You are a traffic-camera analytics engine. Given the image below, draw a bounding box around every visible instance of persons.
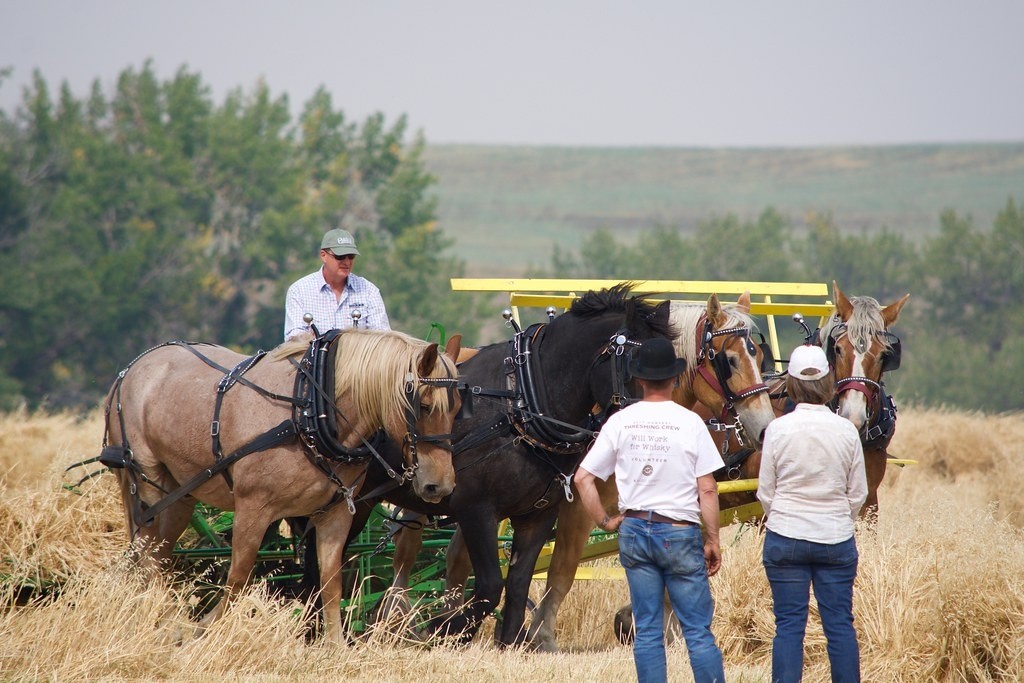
[756,346,868,683]
[285,228,393,343]
[574,339,726,683]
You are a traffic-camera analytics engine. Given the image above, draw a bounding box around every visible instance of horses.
[104,280,912,658]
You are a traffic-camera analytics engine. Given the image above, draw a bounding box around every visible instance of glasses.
[324,250,356,261]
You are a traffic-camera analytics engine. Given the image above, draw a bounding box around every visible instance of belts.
[626,508,695,523]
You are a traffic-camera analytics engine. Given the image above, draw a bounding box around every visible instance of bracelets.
[599,515,610,529]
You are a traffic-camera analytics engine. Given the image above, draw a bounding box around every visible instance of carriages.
[0,278,679,660]
[449,276,919,526]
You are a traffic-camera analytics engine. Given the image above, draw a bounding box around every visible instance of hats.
[787,344,829,381]
[628,339,687,380]
[321,229,361,256]
